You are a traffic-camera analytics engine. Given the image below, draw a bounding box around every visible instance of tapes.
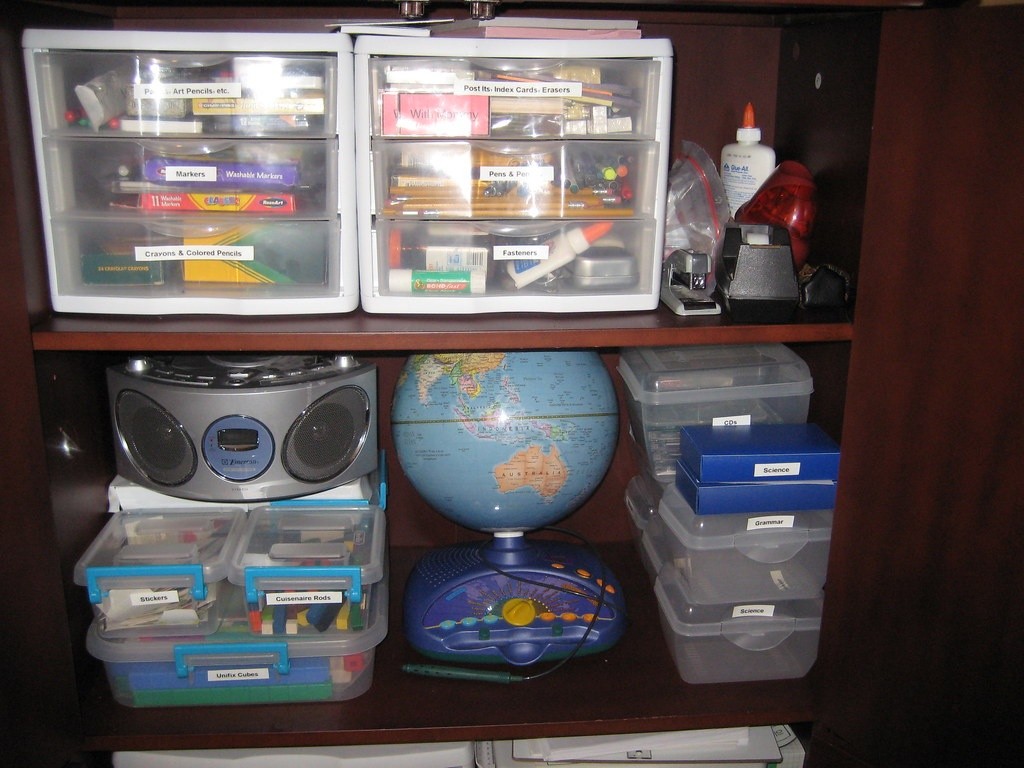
[745,230,770,246]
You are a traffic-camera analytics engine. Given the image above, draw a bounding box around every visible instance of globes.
[389,349,626,667]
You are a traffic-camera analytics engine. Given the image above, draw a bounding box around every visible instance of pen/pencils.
[493,73,614,98]
[483,151,637,205]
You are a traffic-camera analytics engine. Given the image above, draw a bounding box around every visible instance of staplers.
[662,247,724,316]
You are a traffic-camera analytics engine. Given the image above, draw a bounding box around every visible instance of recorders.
[107,353,377,502]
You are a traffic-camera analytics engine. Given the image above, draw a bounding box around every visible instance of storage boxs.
[659,483,832,605]
[370,137,660,220]
[474,723,806,768]
[628,444,670,503]
[354,36,672,316]
[84,566,391,710]
[20,28,358,317]
[44,137,339,220]
[226,507,387,636]
[615,342,815,476]
[674,459,837,514]
[369,55,661,140]
[681,425,840,485]
[73,508,248,637]
[51,220,340,298]
[653,563,827,684]
[377,220,652,298]
[625,478,656,549]
[33,51,336,138]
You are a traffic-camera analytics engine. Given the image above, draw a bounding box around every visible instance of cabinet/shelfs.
[0,0,1024,768]
